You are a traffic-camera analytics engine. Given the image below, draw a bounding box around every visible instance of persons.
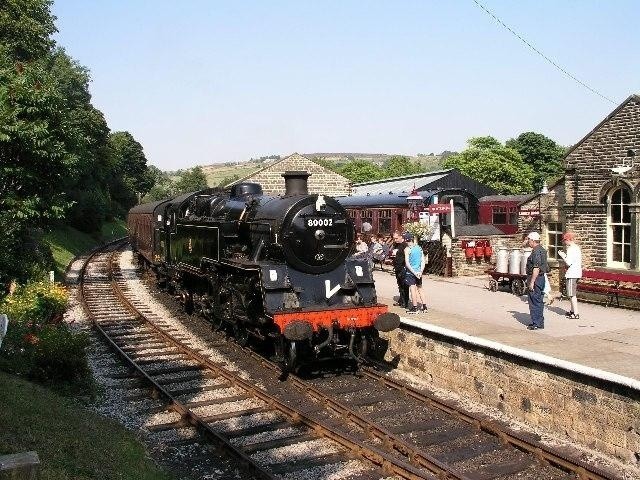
[365,234,387,262]
[356,234,368,258]
[558,232,583,319]
[526,230,548,332]
[404,234,427,314]
[392,231,412,308]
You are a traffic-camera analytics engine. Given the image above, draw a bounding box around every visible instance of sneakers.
[393,302,428,314]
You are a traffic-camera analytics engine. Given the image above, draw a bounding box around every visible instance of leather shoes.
[527,322,544,330]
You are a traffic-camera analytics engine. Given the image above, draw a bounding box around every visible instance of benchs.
[561,267,640,308]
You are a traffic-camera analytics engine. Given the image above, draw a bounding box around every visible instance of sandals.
[565,311,579,319]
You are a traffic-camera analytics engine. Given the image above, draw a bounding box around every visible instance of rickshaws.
[483,268,553,296]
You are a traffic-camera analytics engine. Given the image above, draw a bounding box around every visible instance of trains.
[126,169,402,376]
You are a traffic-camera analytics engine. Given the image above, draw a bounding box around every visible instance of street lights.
[538,181,549,232]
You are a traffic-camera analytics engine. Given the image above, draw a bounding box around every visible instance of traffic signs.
[428,203,451,215]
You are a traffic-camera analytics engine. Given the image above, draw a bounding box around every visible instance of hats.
[560,233,572,242]
[523,232,541,244]
